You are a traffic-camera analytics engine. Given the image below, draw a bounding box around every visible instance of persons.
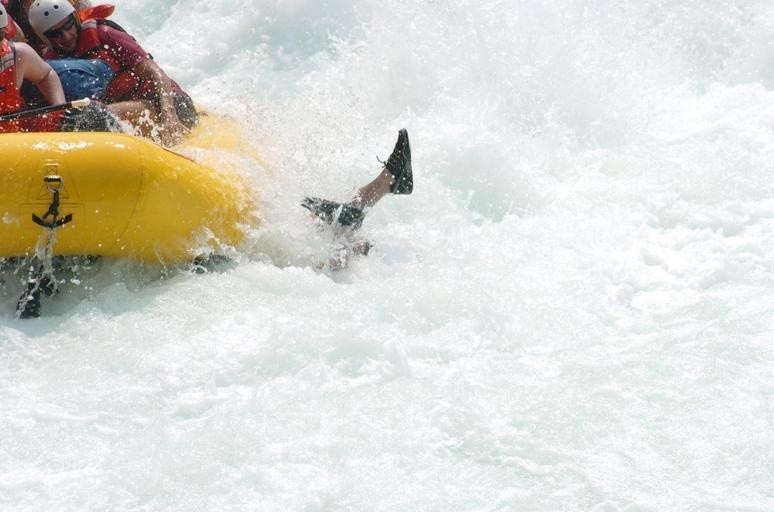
[301,128,414,273]
[0,0,199,148]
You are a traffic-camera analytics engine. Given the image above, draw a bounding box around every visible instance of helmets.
[28,0,79,51]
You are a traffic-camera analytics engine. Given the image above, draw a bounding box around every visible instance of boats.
[0,101,263,267]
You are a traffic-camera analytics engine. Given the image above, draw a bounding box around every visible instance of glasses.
[43,18,76,39]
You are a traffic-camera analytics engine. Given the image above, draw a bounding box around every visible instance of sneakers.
[301,197,364,229]
[381,128,414,195]
